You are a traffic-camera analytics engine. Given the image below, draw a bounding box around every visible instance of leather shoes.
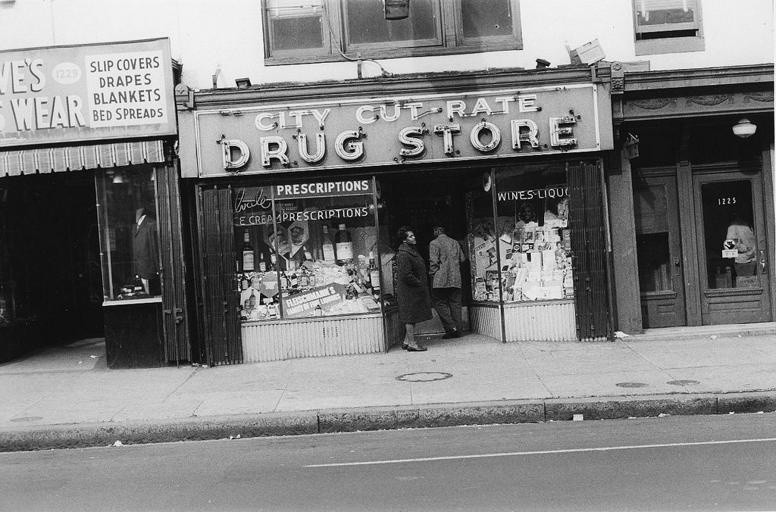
[442,330,465,338]
[402,342,427,351]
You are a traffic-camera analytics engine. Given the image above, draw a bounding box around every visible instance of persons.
[725,202,758,276]
[516,202,539,231]
[543,194,570,229]
[126,207,160,280]
[428,221,468,338]
[393,225,434,351]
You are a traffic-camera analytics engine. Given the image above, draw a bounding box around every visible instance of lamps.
[732,119,757,139]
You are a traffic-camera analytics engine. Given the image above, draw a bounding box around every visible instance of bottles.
[358,250,381,295]
[314,304,322,317]
[249,288,256,307]
[241,272,249,291]
[259,251,267,272]
[267,247,277,265]
[281,268,297,288]
[300,269,308,286]
[302,219,353,265]
[241,225,255,272]
